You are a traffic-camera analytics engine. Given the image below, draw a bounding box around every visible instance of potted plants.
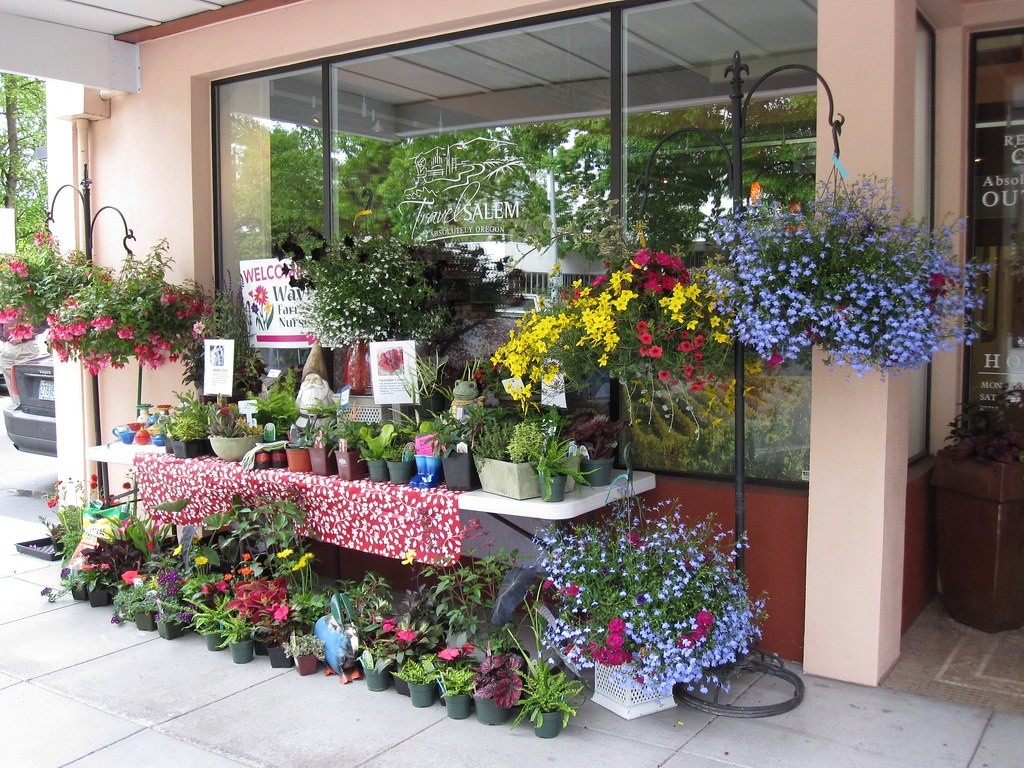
[157,348,633,501]
[934,387,1024,633]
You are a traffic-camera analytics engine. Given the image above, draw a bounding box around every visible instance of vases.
[67,580,677,739]
[0,322,47,346]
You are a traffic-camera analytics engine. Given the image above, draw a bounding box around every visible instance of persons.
[290,373,338,447]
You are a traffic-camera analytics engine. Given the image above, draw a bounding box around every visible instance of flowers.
[38,474,768,697]
[0,172,999,416]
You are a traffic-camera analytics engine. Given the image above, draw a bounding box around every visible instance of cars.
[3,354,57,457]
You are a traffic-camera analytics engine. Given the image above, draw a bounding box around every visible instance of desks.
[82,441,657,683]
